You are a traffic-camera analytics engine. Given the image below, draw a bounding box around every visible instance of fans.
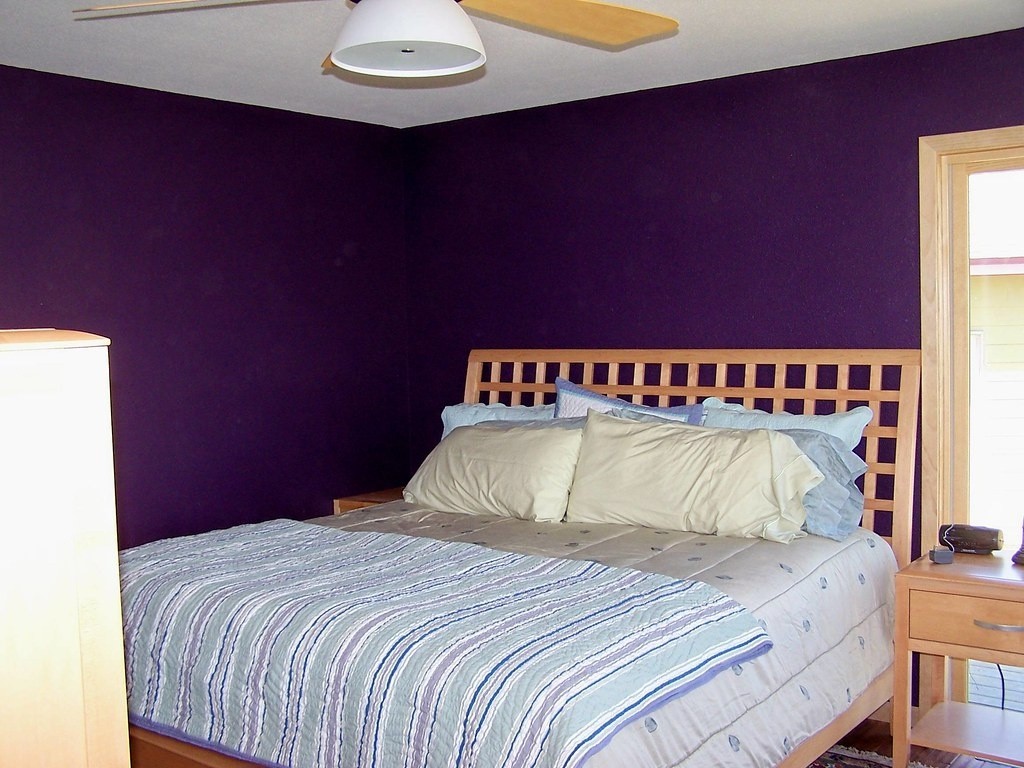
[69,0,682,52]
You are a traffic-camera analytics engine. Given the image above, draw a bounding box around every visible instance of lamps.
[330,0,495,81]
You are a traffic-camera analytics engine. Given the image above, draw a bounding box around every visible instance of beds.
[117,347,920,768]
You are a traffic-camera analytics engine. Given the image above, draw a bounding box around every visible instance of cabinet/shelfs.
[0,328,133,768]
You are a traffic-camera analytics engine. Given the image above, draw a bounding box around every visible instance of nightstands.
[333,488,405,515]
[893,553,1024,768]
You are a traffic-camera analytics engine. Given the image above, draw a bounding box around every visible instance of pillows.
[440,401,554,440]
[478,416,588,431]
[618,408,868,544]
[402,425,583,524]
[703,397,874,453]
[551,377,703,425]
[564,407,822,546]
